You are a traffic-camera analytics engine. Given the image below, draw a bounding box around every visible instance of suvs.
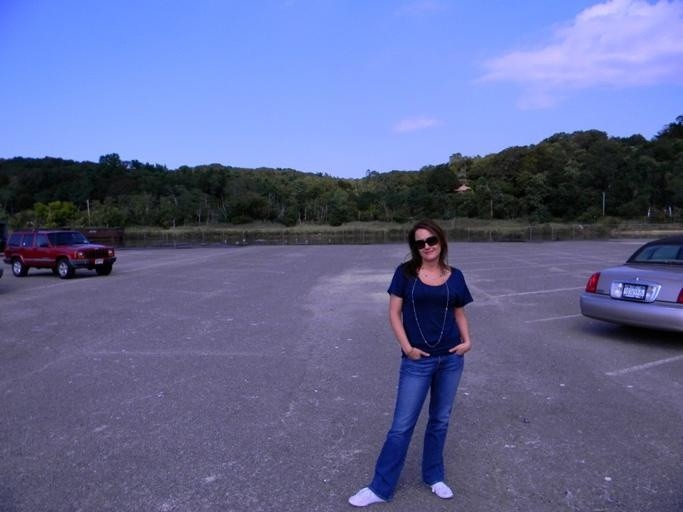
[4,230,117,279]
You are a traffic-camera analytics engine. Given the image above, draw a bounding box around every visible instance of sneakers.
[429,481,454,499]
[348,487,388,507]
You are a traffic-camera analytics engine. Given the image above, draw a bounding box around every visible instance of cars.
[580,235,683,331]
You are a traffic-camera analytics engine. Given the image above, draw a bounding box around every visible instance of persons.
[348,219,474,507]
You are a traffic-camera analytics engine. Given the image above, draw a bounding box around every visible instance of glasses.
[414,236,439,249]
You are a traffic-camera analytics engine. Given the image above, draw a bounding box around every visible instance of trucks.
[0,217,123,253]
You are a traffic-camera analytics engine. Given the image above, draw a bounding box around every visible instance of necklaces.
[419,269,445,281]
